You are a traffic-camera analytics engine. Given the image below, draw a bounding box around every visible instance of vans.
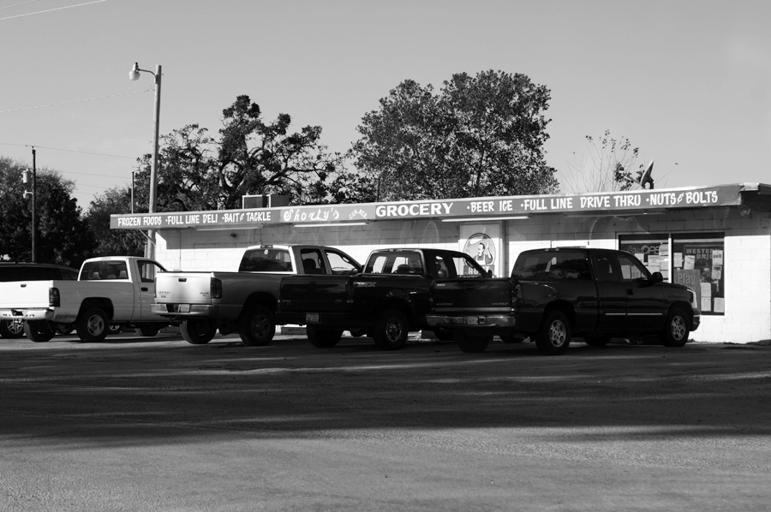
[0,262,85,336]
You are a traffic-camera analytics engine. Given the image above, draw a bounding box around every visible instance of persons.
[472,242,491,265]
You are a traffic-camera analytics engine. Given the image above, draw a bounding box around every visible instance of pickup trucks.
[150,240,366,345]
[275,247,503,352]
[0,255,180,342]
[425,243,705,353]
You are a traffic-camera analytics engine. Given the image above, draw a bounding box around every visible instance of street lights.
[126,59,166,279]
[19,167,38,262]
